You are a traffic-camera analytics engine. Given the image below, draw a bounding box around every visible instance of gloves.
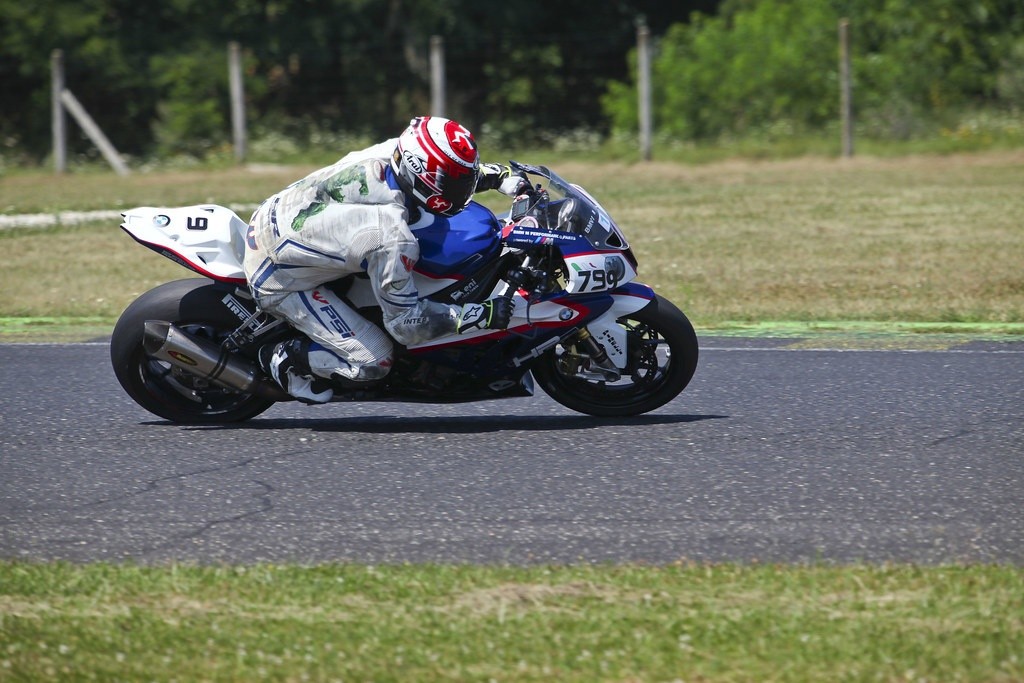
[476,163,534,199]
[456,296,515,334]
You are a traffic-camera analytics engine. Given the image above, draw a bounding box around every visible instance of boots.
[258,336,333,405]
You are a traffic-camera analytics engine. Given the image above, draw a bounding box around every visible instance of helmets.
[390,116,481,218]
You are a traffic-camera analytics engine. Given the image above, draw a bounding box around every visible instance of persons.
[242,117,535,415]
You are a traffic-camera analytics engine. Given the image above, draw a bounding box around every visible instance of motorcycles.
[108,159,701,421]
